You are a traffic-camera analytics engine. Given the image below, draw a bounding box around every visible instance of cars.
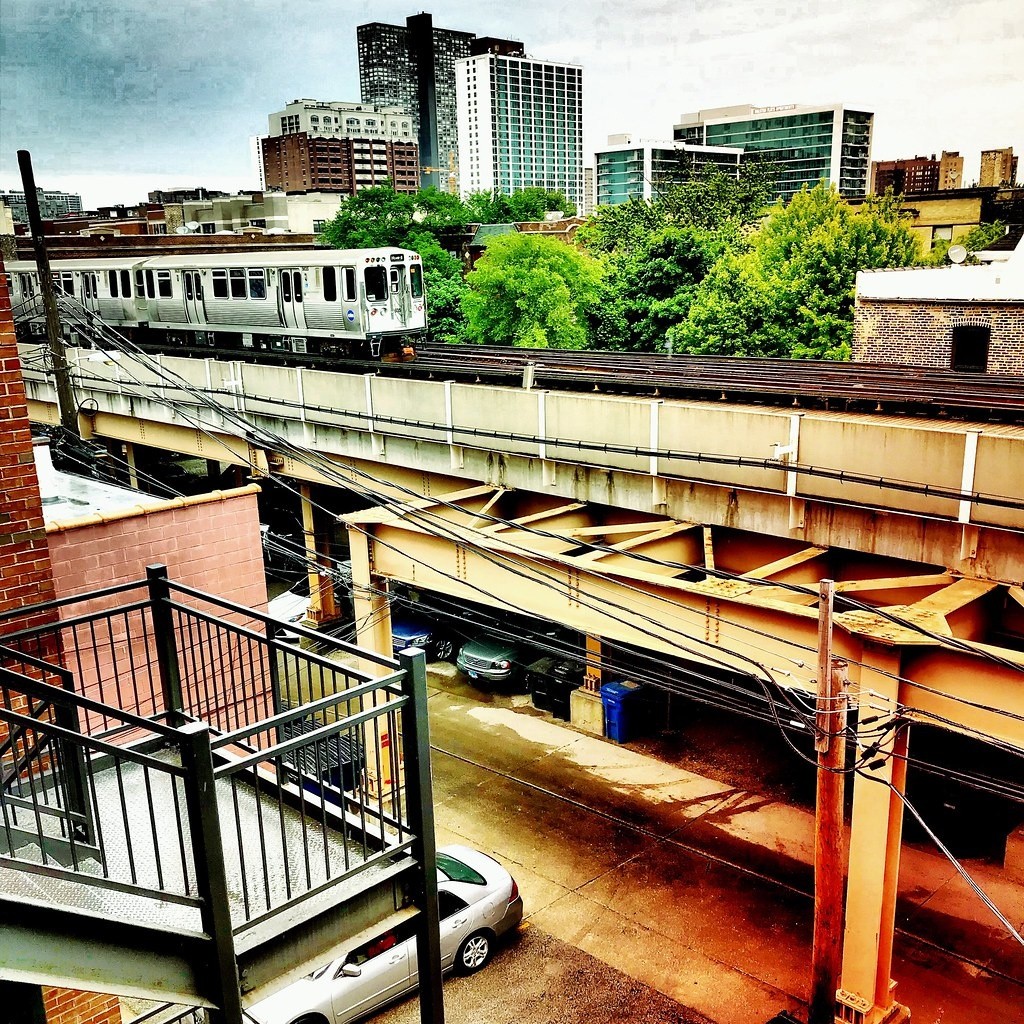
[268,561,351,640]
[266,559,586,722]
[241,845,524,1024]
[392,617,466,662]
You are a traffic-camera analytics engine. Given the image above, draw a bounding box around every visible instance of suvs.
[457,634,542,694]
[524,647,596,715]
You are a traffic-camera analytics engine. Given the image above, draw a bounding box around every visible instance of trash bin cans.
[598,678,647,745]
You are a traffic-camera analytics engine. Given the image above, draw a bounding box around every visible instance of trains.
[0,247,427,355]
